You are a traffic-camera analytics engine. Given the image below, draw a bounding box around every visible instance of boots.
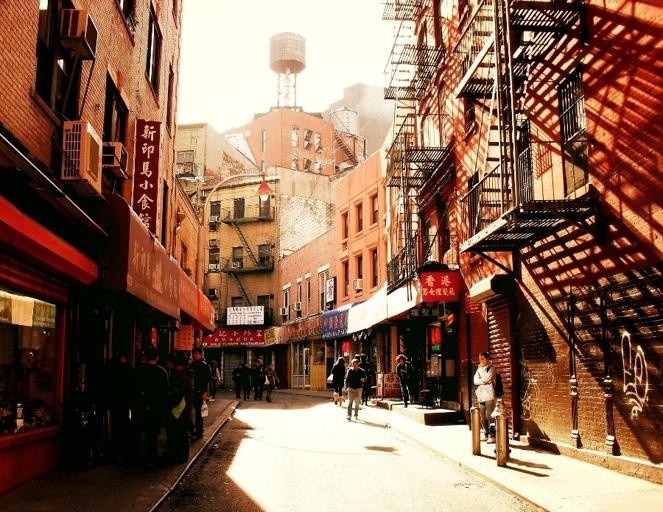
[338,396,345,406]
[334,392,338,404]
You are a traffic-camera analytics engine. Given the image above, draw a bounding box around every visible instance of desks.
[375,372,401,400]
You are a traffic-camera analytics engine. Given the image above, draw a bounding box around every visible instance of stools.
[420,389,432,408]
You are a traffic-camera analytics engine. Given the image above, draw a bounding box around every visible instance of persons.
[92,346,221,476]
[396,354,417,407]
[232,359,279,403]
[331,354,374,421]
[474,351,498,443]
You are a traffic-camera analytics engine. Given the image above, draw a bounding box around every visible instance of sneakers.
[487,437,496,443]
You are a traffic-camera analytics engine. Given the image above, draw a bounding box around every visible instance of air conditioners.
[60,9,98,60]
[294,302,301,311]
[61,121,103,196]
[280,307,288,316]
[207,216,240,297]
[353,279,363,291]
[103,142,129,180]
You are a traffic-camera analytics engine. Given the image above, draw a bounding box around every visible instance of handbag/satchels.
[327,374,333,384]
[494,373,504,397]
